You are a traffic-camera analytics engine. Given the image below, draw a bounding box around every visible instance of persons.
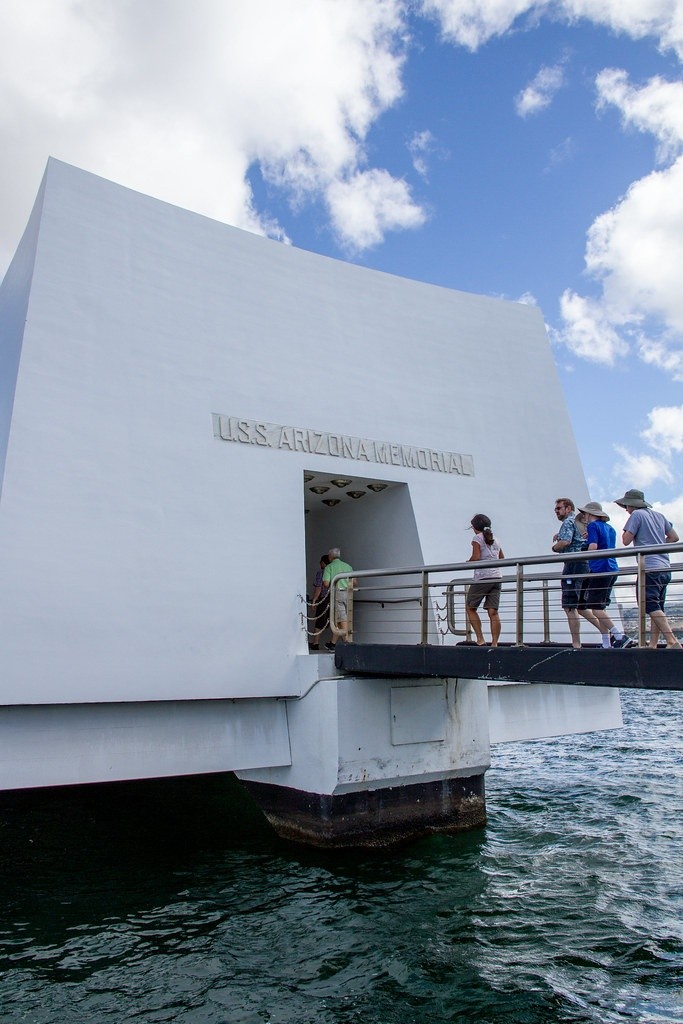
[552,498,633,649]
[466,515,505,647]
[614,489,683,649]
[309,548,357,653]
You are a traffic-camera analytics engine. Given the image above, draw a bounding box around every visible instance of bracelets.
[553,547,557,552]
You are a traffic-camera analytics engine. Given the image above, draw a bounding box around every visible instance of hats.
[321,554,330,564]
[308,642,319,650]
[614,489,653,508]
[577,502,610,521]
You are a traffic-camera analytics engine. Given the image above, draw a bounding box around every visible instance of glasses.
[555,506,567,510]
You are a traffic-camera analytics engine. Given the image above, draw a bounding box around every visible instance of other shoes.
[609,635,632,648]
[570,645,583,649]
[665,641,683,649]
[596,645,612,649]
[470,642,487,646]
[324,641,336,653]
